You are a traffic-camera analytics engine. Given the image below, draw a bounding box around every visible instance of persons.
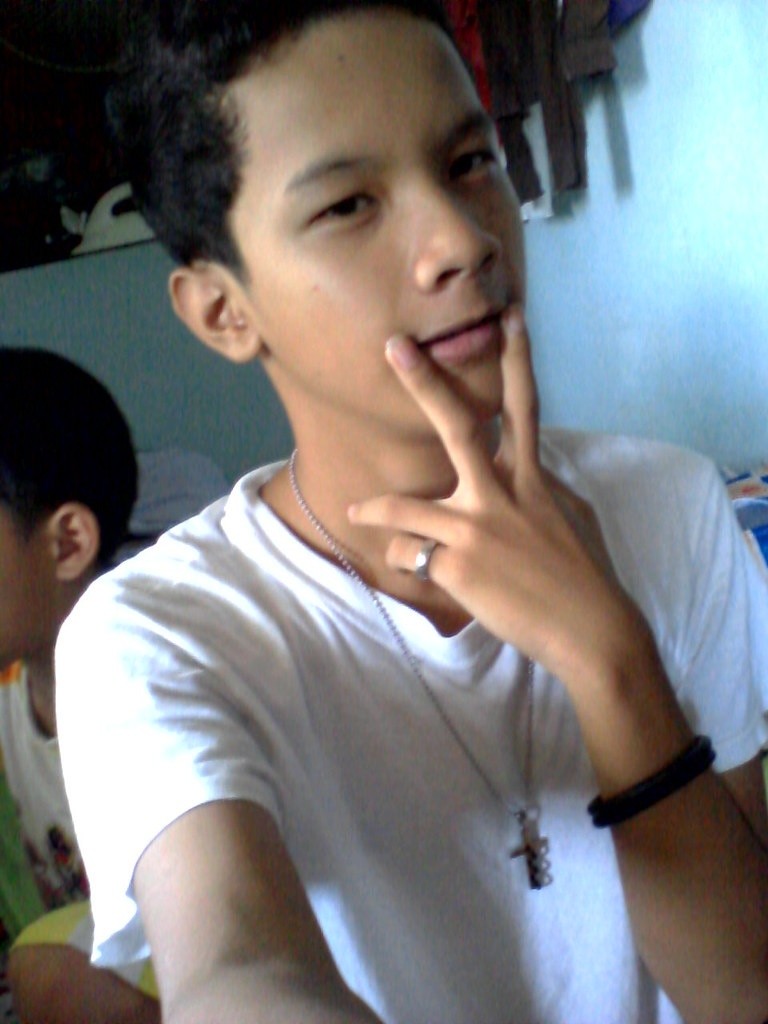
[0,346,160,1024]
[51,0,766,1024]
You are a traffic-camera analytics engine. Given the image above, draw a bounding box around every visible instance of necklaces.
[290,447,538,890]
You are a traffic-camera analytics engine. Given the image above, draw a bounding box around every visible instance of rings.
[415,541,436,582]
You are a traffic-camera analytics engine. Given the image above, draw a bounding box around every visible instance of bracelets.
[587,737,715,828]
